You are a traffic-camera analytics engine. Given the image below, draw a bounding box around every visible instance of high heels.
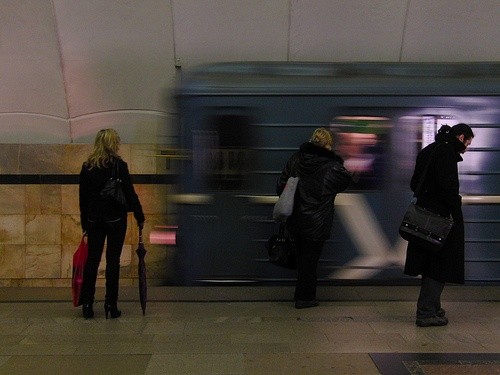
[105,303,122,318]
[83,304,94,320]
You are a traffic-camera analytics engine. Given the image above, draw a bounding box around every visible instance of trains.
[169,61,500,285]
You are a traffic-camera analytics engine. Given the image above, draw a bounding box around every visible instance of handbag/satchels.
[399,201,454,251]
[273,152,301,218]
[266,217,294,268]
[72,233,89,306]
[100,157,136,212]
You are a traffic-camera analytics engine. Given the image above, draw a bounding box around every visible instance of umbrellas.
[136,224,147,315]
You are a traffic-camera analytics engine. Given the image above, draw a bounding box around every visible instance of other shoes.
[437,309,446,316]
[416,315,448,327]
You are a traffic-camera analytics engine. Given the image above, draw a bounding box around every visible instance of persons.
[79,128,146,320]
[403,123,474,326]
[280,127,351,309]
